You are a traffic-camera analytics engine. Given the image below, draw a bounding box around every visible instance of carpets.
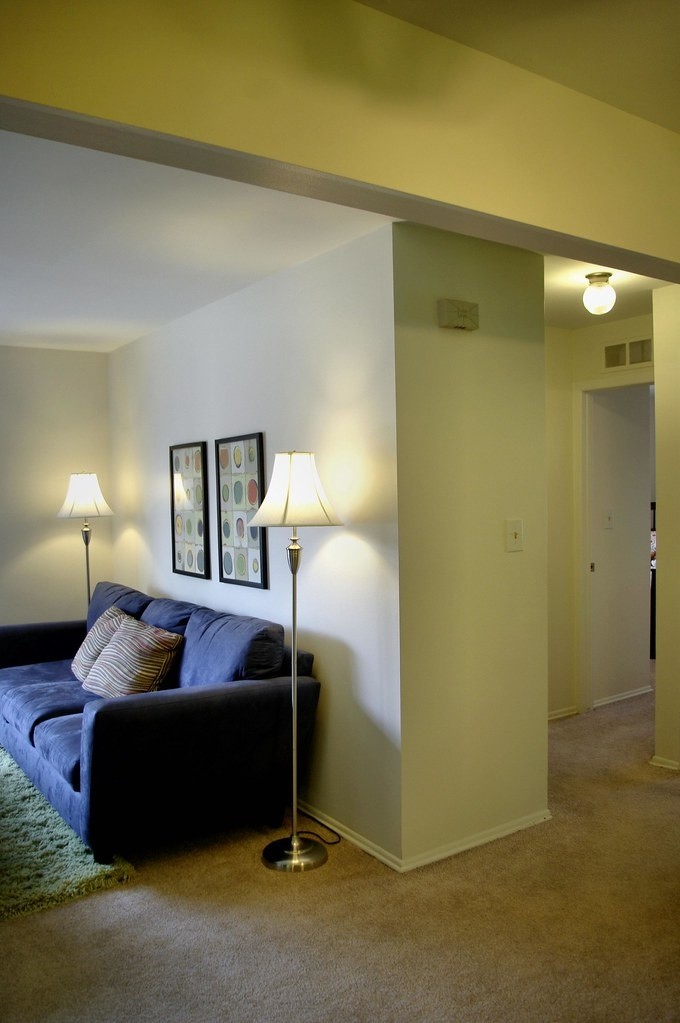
[0,744,141,922]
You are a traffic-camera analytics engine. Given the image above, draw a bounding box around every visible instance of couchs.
[0,581,321,868]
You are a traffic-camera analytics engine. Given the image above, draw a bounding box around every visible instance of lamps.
[247,450,344,871]
[582,272,617,315]
[55,471,115,605]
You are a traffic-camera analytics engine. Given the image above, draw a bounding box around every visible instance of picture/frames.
[84,615,183,699]
[215,432,269,590]
[168,441,211,580]
[71,605,127,683]
